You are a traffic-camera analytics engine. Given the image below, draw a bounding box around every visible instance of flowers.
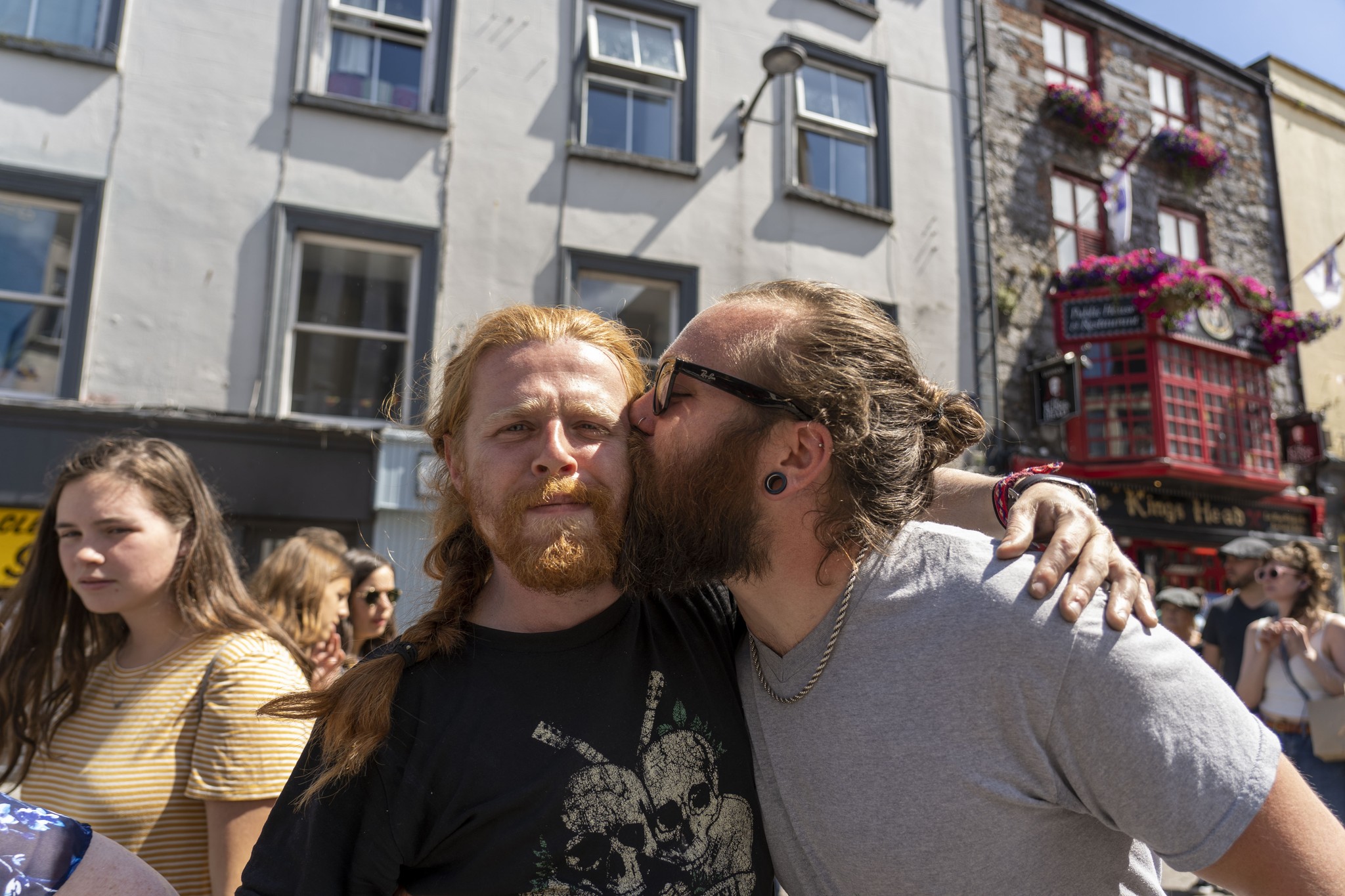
[1043,81,1124,148]
[1053,247,1337,358]
[1155,123,1229,179]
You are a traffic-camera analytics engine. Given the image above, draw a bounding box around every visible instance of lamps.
[734,43,802,158]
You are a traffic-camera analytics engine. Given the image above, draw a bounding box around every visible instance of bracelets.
[1009,473,1098,515]
[992,462,1063,552]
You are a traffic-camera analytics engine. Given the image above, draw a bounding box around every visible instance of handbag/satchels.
[1309,694,1345,762]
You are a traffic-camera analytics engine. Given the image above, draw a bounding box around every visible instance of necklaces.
[750,538,868,704]
[110,622,188,709]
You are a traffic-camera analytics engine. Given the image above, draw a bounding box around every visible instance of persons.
[625,279,1345,896]
[0,438,317,896]
[1138,537,1345,831]
[233,309,1164,896]
[242,526,399,691]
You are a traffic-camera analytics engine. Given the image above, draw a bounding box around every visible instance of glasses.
[653,359,829,421]
[1254,565,1304,584]
[365,590,398,604]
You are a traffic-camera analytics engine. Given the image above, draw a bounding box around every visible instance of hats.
[1155,587,1200,615]
[1220,536,1272,559]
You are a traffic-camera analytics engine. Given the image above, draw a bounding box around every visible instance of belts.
[1262,721,1310,733]
